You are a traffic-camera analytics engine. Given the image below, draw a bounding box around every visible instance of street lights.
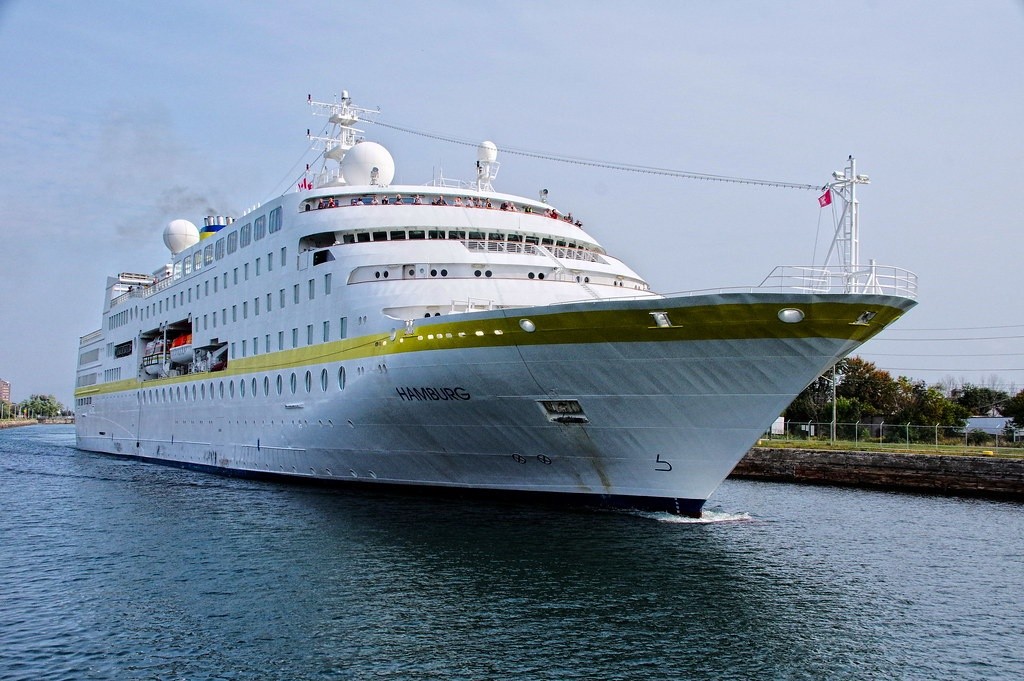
[2,402,36,419]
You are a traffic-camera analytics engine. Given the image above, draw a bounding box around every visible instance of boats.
[73,88,921,519]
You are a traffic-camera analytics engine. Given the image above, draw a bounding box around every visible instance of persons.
[382,195,389,205]
[318,198,325,209]
[127,281,156,292]
[575,219,582,228]
[394,193,404,205]
[432,196,448,206]
[455,196,493,209]
[500,200,532,214]
[542,209,558,220]
[327,197,336,208]
[352,198,364,206]
[371,196,378,205]
[562,212,573,224]
[412,194,423,205]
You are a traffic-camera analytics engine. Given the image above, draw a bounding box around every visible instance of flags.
[818,188,832,208]
[298,178,312,190]
